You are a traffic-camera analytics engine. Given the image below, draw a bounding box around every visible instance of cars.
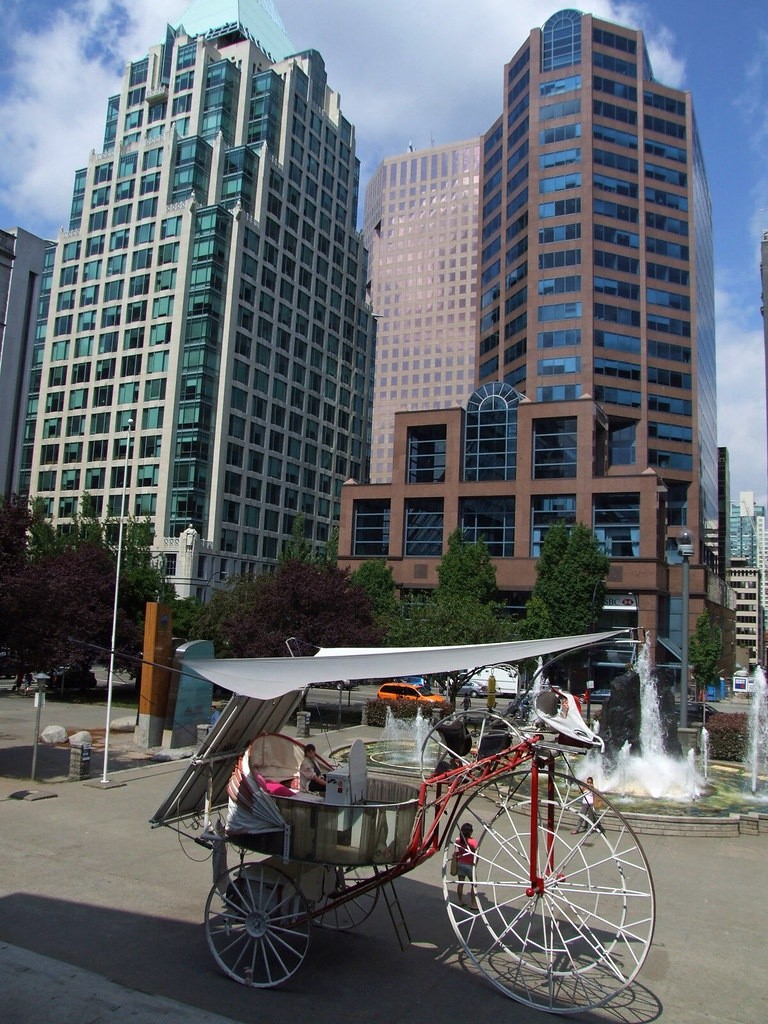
[676,701,721,723]
[310,679,360,691]
[438,681,489,698]
[518,684,562,702]
[578,689,611,705]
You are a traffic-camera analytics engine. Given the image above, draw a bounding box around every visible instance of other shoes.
[571,830,578,834]
[470,906,484,910]
[458,902,467,907]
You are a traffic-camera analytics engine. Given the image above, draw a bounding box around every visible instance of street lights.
[676,527,696,730]
[202,570,227,605]
[30,672,52,782]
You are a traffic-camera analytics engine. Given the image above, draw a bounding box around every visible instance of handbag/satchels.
[450,841,459,876]
[594,794,602,808]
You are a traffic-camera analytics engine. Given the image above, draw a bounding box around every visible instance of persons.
[454,823,484,910]
[460,693,472,711]
[298,744,342,793]
[209,706,220,725]
[15,670,34,696]
[572,776,596,835]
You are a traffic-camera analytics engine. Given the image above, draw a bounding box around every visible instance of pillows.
[266,779,296,796]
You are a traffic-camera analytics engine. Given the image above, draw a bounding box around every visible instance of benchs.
[260,776,324,803]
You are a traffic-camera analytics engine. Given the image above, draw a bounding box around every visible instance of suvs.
[403,677,432,691]
[377,683,447,705]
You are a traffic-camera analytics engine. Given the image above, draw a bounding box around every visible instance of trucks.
[458,663,519,698]
